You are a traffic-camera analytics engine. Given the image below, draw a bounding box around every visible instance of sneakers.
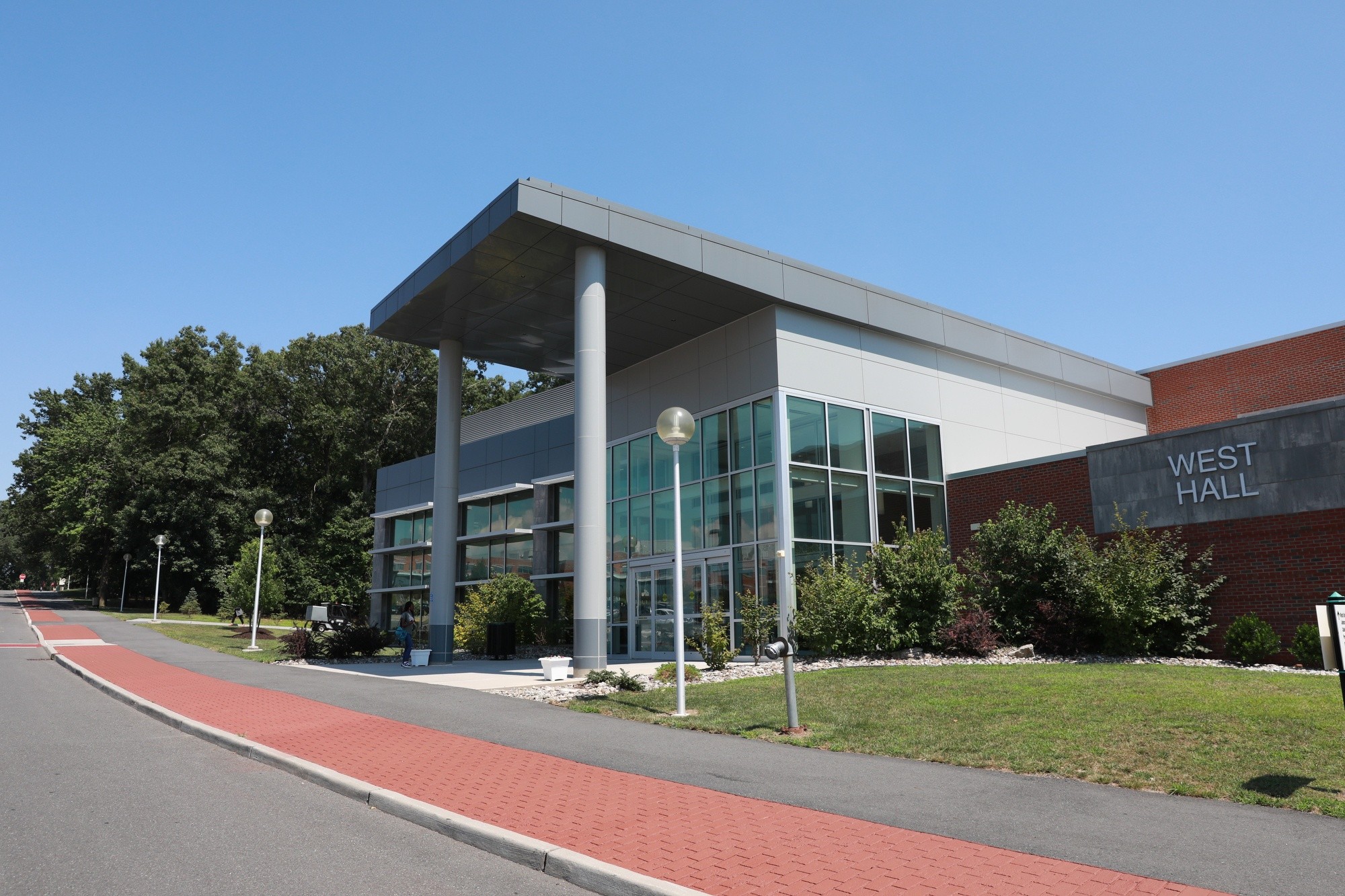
[401,662,412,668]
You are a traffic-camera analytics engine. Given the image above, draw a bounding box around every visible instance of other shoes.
[242,624,245,627]
[229,623,233,626]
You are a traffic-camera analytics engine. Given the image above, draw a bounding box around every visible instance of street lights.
[120,554,131,612]
[152,535,167,621]
[242,509,274,651]
[656,406,696,717]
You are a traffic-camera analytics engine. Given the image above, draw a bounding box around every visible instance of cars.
[654,608,693,631]
[607,608,619,618]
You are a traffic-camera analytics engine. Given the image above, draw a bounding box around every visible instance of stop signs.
[20,574,26,579]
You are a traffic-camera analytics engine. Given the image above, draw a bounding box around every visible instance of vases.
[538,655,572,681]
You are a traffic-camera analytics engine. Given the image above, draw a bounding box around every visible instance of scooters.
[306,602,353,632]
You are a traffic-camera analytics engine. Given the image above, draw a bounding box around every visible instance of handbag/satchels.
[51,583,54,587]
[235,608,244,616]
[395,626,408,642]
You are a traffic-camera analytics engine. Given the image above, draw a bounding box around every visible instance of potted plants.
[410,622,433,667]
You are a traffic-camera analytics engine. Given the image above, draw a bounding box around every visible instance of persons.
[400,601,415,668]
